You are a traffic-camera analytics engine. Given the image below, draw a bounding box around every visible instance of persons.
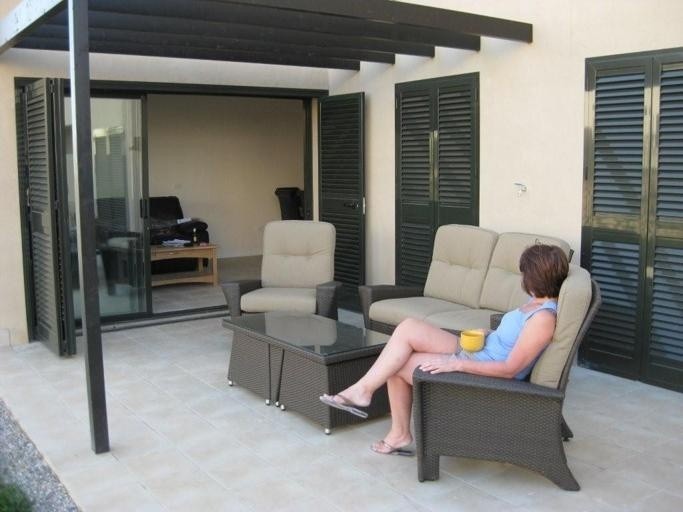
[316,242,568,455]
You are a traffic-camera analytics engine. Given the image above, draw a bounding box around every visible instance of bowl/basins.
[460,330,485,352]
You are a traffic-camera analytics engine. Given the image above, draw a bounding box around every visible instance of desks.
[150,245,220,287]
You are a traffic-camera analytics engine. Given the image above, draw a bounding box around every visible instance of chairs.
[222,220,345,319]
[95,195,210,295]
[409,263,603,491]
[274,186,304,219]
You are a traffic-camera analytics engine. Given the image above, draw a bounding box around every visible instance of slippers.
[371,440,415,456]
[319,393,369,419]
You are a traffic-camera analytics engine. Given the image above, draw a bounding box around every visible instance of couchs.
[358,222,574,339]
